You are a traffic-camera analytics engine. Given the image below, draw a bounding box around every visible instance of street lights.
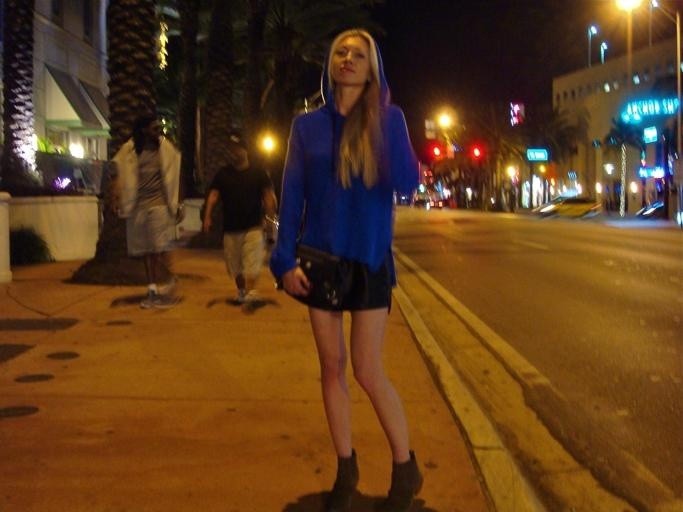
[615,0,642,98]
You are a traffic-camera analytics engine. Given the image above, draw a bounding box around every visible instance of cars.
[533,195,601,217]
[638,202,666,218]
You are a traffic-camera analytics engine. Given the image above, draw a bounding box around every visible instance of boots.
[326,447,358,512]
[384,450,423,512]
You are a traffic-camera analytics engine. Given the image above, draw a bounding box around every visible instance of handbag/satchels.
[279,243,352,309]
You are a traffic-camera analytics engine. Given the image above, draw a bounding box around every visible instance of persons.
[204,133,278,302]
[268,29,423,512]
[109,113,186,309]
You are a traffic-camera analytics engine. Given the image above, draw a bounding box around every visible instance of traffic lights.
[438,111,455,129]
[431,142,445,160]
[469,144,484,159]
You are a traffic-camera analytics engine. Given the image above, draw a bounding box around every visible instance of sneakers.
[238,288,261,304]
[141,276,183,308]
